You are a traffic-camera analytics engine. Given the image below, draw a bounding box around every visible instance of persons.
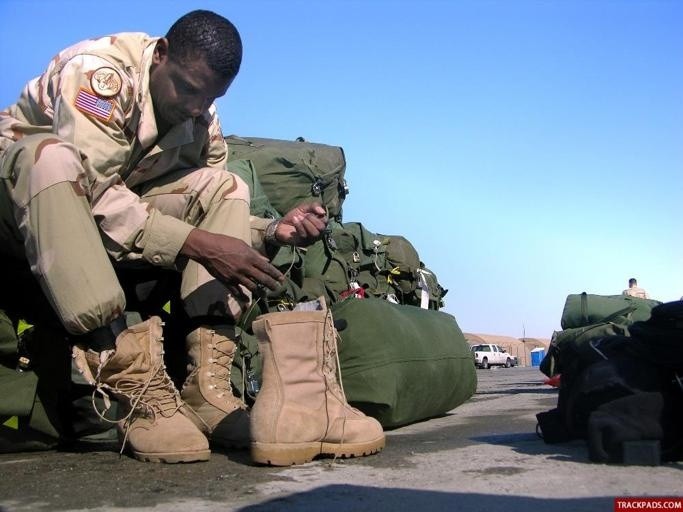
[0,9,329,464]
[622,278,649,300]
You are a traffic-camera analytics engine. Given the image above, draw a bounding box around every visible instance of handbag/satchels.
[537,292,682,464]
[224,135,478,425]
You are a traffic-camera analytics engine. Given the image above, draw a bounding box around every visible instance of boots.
[70,316,212,464]
[248,310,386,466]
[181,326,252,446]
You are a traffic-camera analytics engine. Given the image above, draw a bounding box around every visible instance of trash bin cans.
[531,351,545,367]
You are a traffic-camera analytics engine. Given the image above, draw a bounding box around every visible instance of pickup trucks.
[471,344,512,369]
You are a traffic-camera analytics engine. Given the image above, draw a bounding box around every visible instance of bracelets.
[265,219,282,247]
